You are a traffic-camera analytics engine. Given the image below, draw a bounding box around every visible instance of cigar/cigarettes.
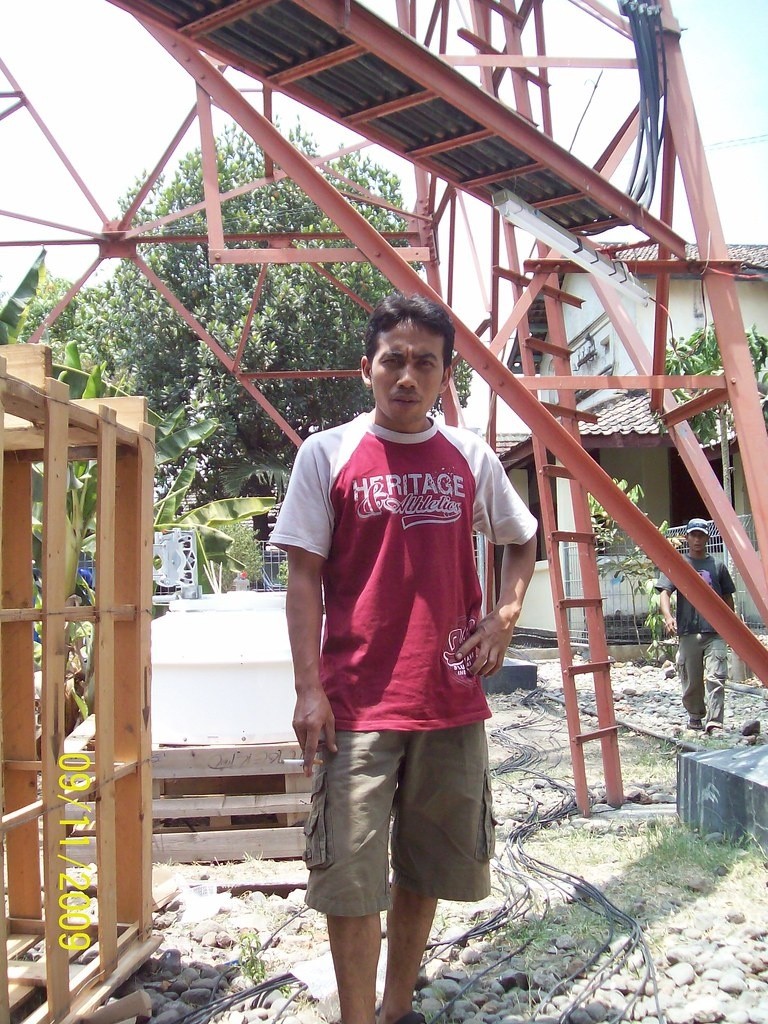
[278,758,323,766]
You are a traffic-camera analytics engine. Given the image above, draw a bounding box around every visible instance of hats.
[686,518,710,535]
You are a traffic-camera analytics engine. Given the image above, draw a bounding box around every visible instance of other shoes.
[688,718,703,729]
[709,726,725,739]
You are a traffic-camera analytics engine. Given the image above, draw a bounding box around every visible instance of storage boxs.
[61,712,317,872]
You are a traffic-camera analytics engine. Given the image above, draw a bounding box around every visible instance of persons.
[655,519,737,740]
[269,292,538,1024]
[249,471,285,588]
